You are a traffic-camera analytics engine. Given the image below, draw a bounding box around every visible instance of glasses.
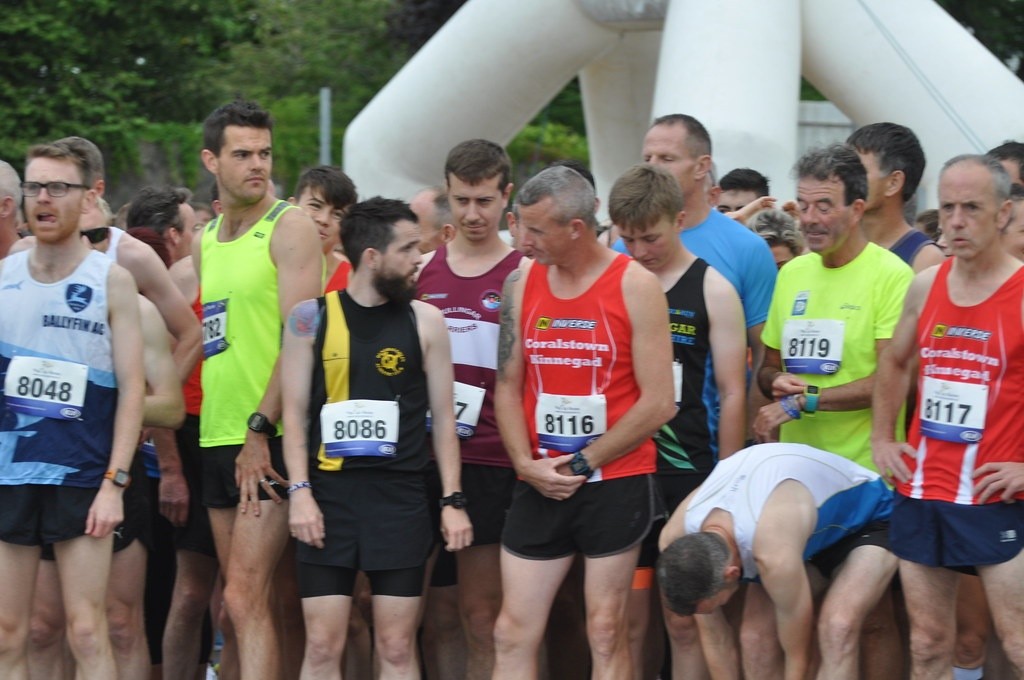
[20,182,90,197]
[80,227,110,243]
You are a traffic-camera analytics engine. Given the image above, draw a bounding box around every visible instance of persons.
[0,102,1024,680]
